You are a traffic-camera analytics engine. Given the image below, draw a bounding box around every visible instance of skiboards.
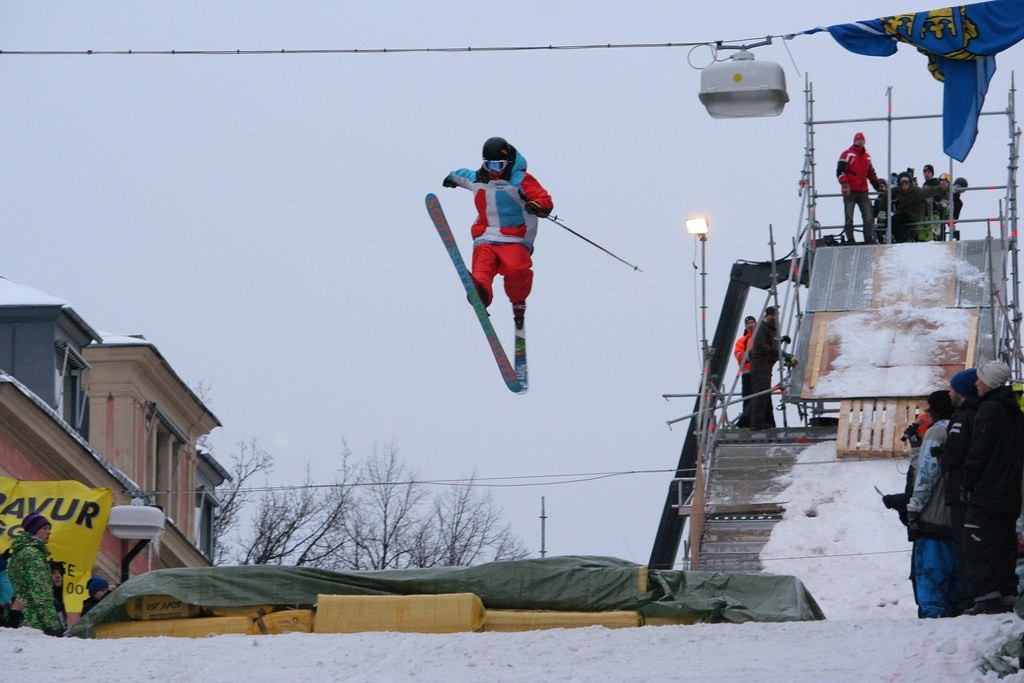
[425,192,529,395]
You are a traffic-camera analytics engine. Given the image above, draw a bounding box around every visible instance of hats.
[86,576,108,590]
[914,361,1011,433]
[854,132,865,140]
[745,316,754,322]
[22,511,52,535]
[893,164,967,193]
[766,306,779,316]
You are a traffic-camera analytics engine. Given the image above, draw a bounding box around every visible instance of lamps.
[698,40,790,122]
[105,492,166,543]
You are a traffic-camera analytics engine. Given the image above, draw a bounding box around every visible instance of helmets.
[482,137,509,159]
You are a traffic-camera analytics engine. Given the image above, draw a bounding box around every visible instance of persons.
[837,132,884,245]
[882,360,1024,621]
[735,306,799,431]
[442,137,554,330]
[0,512,116,638]
[872,165,968,244]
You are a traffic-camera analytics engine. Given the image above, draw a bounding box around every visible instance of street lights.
[685,217,710,381]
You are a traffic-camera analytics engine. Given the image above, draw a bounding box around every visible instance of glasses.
[88,589,99,596]
[482,160,507,171]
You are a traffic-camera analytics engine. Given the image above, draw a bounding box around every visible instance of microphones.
[900,417,924,442]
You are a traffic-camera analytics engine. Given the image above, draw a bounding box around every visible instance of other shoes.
[512,301,526,317]
[937,585,1018,614]
[467,286,488,309]
[848,238,878,246]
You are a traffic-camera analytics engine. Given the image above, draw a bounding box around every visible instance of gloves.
[944,483,971,505]
[842,183,851,198]
[524,200,541,214]
[900,511,920,530]
[883,495,896,509]
[904,423,922,447]
[44,627,64,638]
[442,176,457,189]
